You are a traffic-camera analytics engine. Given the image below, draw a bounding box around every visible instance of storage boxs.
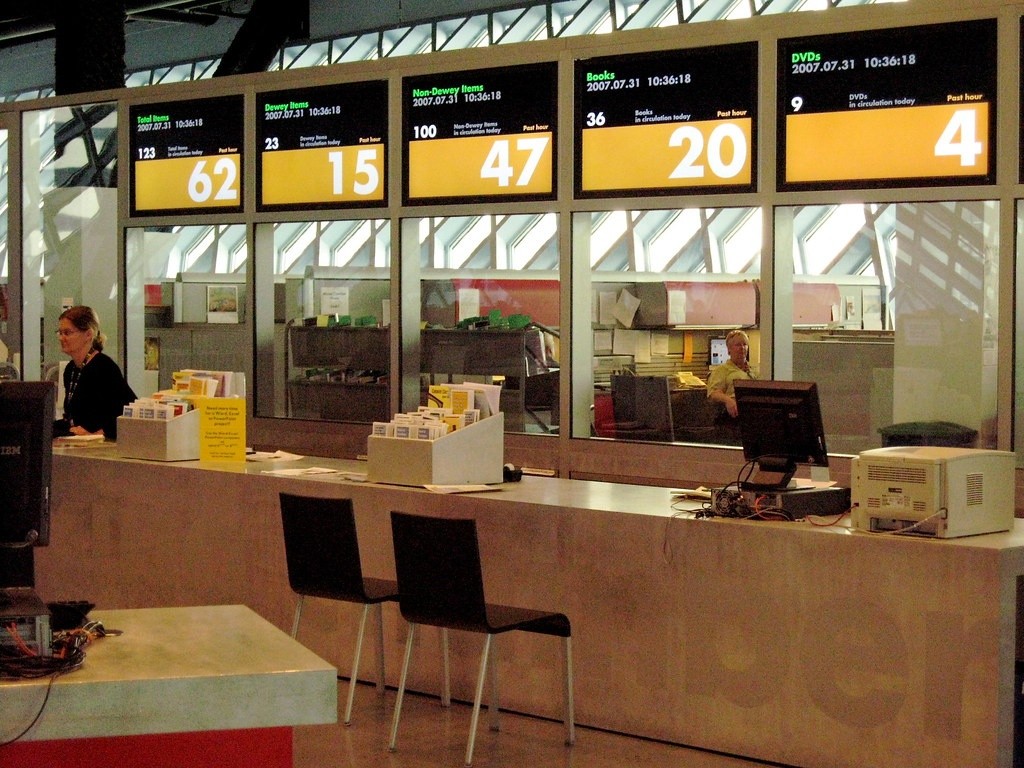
[366,412,503,486]
[115,407,198,463]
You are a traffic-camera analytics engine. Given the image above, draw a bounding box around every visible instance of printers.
[850,446,1015,539]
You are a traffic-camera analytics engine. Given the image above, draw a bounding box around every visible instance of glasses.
[55,328,81,336]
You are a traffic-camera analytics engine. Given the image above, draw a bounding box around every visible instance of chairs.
[387,509,575,764]
[277,490,452,728]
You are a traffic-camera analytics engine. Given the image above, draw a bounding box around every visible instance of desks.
[0,603,338,768]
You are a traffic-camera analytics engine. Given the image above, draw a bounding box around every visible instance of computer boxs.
[711,484,851,521]
[0,588,53,660]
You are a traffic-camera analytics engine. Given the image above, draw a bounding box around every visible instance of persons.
[708,330,763,418]
[53,305,138,444]
[541,331,560,427]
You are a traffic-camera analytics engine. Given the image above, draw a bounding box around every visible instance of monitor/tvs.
[0,381,55,611]
[708,335,749,367]
[734,378,829,492]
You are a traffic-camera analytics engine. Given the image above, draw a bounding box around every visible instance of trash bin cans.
[877,419,981,447]
